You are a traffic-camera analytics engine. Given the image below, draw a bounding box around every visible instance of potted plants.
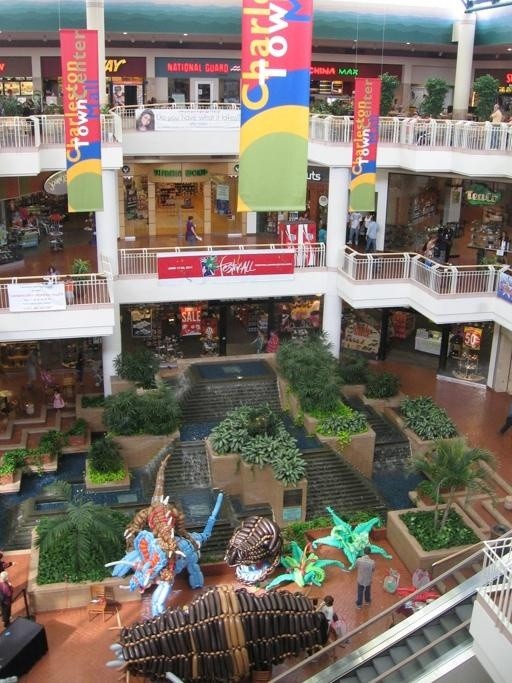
[71,257,92,298]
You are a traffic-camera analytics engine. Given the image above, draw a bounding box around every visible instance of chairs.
[87,583,110,623]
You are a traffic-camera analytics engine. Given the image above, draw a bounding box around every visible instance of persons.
[64,273,75,304]
[51,389,65,414]
[0,552,15,574]
[135,108,155,132]
[74,351,86,387]
[22,348,43,392]
[489,103,504,123]
[497,230,509,265]
[353,545,376,609]
[44,368,57,388]
[44,264,60,283]
[329,614,351,648]
[493,405,512,436]
[0,571,14,628]
[185,215,202,251]
[95,365,104,387]
[249,328,265,354]
[315,595,335,623]
[264,331,280,353]
[298,209,455,261]
